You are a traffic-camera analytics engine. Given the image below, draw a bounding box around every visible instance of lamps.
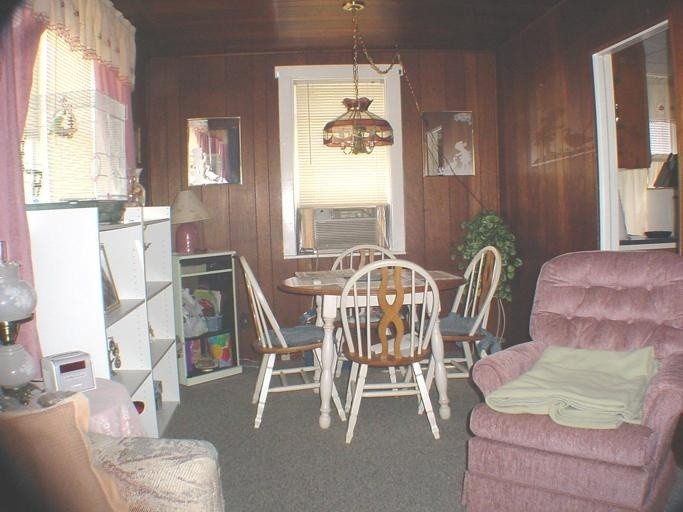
[321,1,394,156]
[0,237,39,406]
[168,189,212,255]
[652,152,680,239]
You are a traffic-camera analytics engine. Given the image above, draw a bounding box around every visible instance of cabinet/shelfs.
[174,249,242,387]
[26,205,184,440]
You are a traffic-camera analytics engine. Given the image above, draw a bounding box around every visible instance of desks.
[0,374,145,438]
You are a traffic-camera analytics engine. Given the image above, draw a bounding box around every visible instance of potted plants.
[450,210,523,304]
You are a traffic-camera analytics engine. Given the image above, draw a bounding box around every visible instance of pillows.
[1,392,131,511]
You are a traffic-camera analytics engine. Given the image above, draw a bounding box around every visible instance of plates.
[645,230,672,239]
[36,390,74,407]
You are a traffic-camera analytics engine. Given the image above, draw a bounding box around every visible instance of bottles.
[124,168,146,206]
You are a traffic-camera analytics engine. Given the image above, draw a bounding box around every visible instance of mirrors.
[184,115,242,188]
[420,109,475,178]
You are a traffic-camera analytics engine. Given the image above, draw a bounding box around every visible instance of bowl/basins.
[77,199,128,224]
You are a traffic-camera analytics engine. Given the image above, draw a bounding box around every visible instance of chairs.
[241,244,502,443]
[462,248,681,512]
[0,394,226,511]
